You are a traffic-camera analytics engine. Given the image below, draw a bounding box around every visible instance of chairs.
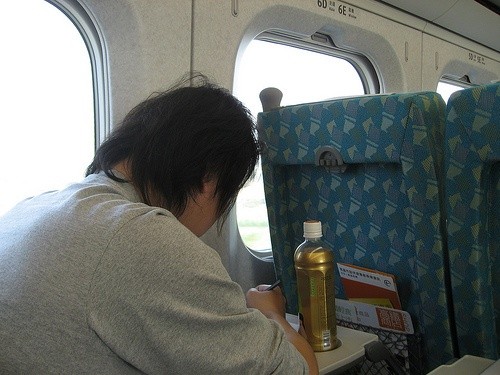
[445,77,500,360]
[256,89,456,374]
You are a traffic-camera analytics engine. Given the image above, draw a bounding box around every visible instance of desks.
[423,353,499,375]
[284,312,407,375]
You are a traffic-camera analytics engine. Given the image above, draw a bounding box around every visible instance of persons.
[0,69,320,375]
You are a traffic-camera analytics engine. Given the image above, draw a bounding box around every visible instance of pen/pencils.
[265,279,282,290]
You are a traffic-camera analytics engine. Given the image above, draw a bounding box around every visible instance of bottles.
[294,220,338,351]
